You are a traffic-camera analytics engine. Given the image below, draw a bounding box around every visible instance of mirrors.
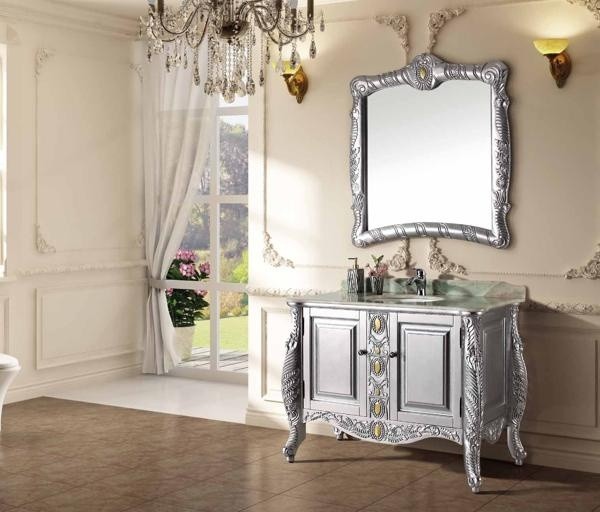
[349,52,513,250]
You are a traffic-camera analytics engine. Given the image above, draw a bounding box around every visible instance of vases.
[165,326,195,362]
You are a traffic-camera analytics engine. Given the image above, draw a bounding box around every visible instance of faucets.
[408,267,427,297]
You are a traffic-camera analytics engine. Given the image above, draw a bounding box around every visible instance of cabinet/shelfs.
[299,303,514,430]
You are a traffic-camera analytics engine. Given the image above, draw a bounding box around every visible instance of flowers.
[165,248,211,327]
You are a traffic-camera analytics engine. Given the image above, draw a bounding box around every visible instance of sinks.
[363,293,445,304]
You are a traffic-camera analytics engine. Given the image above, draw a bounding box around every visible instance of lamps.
[271,57,308,104]
[532,39,571,88]
[136,0,325,103]
